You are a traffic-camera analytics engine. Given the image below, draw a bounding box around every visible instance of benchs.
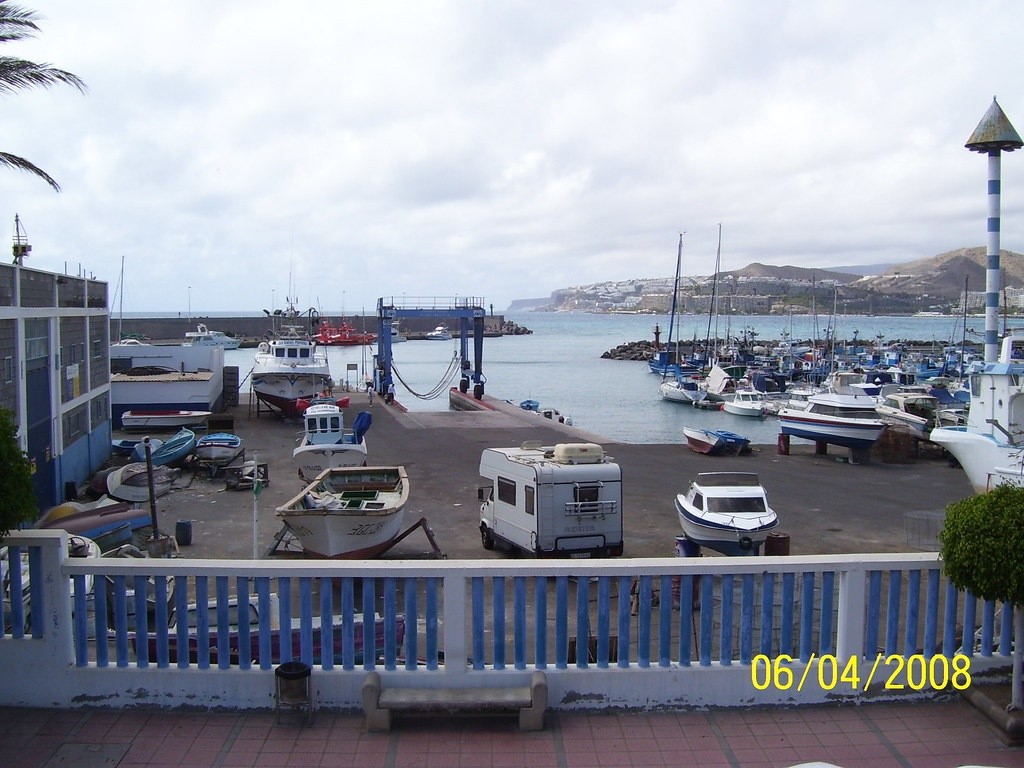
[361,671,548,732]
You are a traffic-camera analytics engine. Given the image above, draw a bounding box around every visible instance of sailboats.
[656,222,985,417]
[108,254,149,346]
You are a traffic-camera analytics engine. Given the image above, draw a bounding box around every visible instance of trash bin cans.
[65,480,77,502]
[278,662,309,705]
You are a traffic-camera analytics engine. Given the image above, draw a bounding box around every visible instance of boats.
[181,324,243,350]
[195,433,241,467]
[504,397,572,427]
[777,288,892,449]
[121,409,213,428]
[425,321,452,340]
[276,464,409,558]
[293,369,368,485]
[874,389,971,443]
[133,589,404,664]
[682,425,751,456]
[649,347,700,378]
[251,226,330,413]
[675,471,780,557]
[0,426,195,620]
[316,290,376,345]
[389,320,407,343]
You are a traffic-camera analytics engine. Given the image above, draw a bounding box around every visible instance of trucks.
[476,440,622,559]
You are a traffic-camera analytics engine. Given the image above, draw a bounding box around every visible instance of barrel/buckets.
[675,536,700,557]
[176,520,192,544]
[764,531,790,556]
[778,433,790,454]
[816,441,827,454]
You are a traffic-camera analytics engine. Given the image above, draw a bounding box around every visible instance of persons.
[367,383,374,408]
[388,384,396,405]
